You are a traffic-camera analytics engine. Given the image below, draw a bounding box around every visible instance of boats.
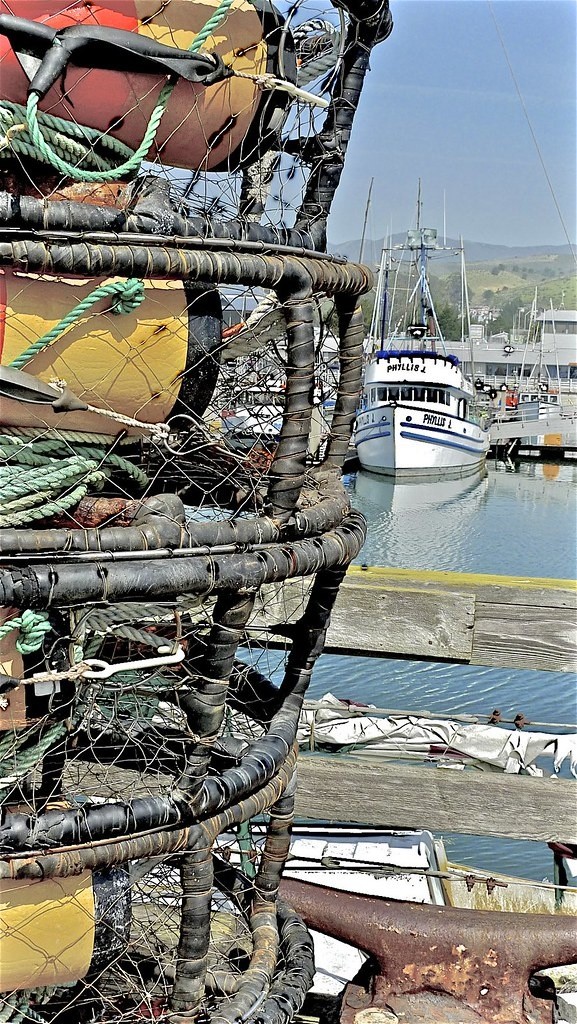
[352,176,493,479]
[0,0,577,1024]
